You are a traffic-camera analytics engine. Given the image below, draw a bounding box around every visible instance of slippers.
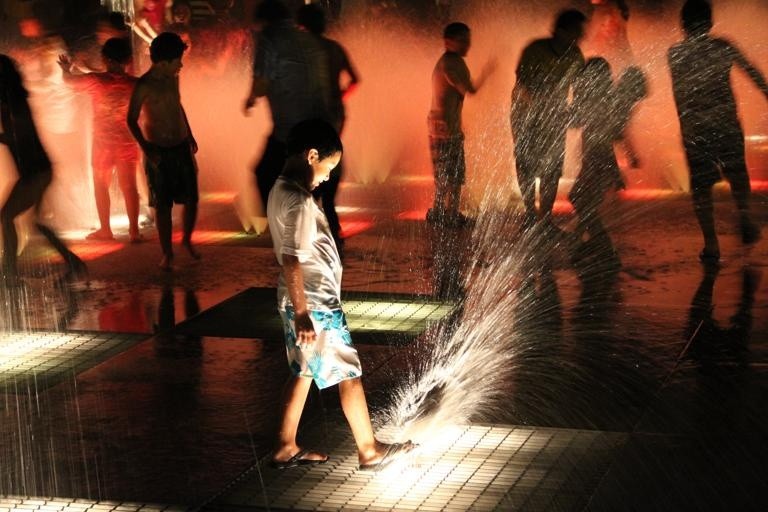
[269,446,331,472]
[358,439,421,473]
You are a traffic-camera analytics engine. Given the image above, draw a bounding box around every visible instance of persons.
[582,0,641,170]
[664,0,766,263]
[424,21,500,230]
[295,2,360,140]
[240,0,343,263]
[507,5,592,220]
[0,1,265,134]
[126,32,201,272]
[264,118,420,473]
[0,52,88,292]
[559,55,643,253]
[56,36,145,243]
